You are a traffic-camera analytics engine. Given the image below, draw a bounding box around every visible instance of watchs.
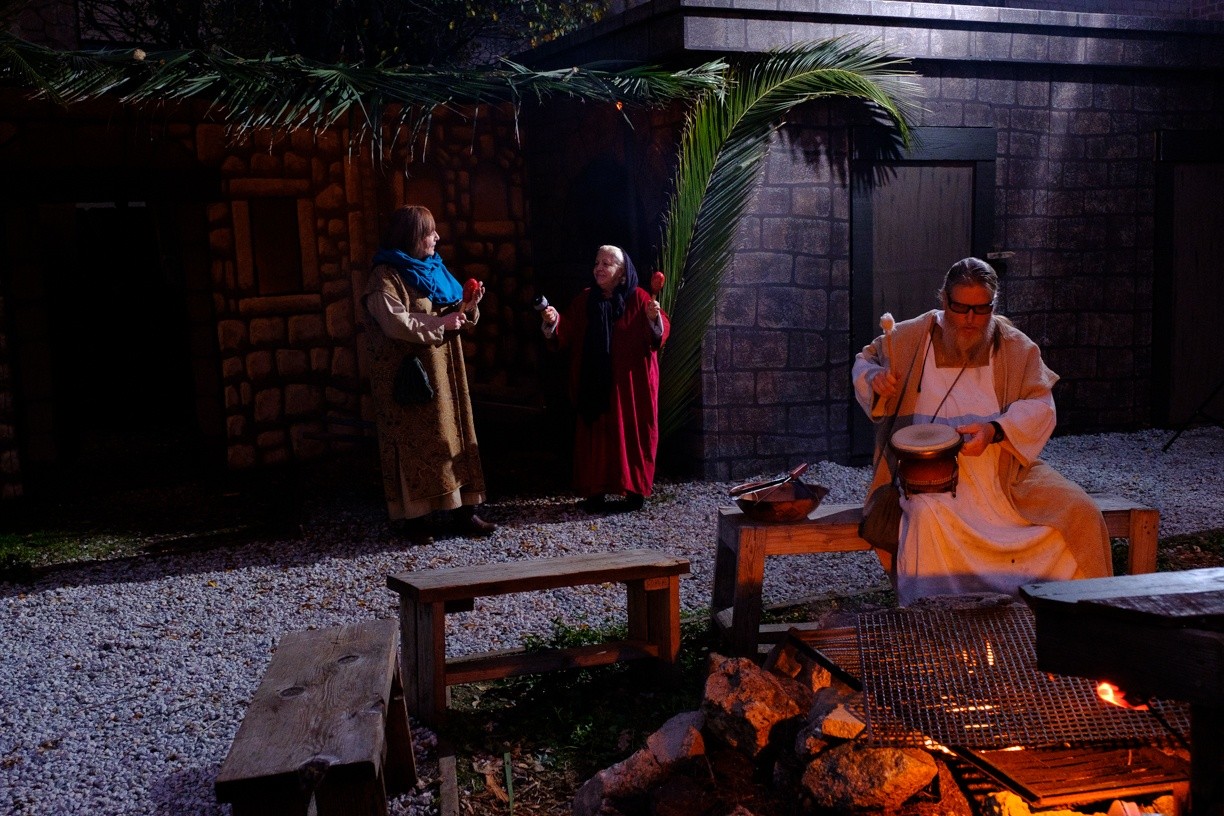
[986,422,1004,443]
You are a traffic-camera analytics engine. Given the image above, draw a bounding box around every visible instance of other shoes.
[574,495,605,511]
[617,492,644,512]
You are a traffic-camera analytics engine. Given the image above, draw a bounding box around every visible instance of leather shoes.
[454,511,499,535]
[404,518,434,545]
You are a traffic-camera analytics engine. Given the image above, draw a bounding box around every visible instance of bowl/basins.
[729,477,831,521]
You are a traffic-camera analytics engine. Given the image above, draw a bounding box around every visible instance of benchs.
[215,619,418,816]
[387,550,691,732]
[710,497,1161,670]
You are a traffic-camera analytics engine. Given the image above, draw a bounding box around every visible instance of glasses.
[947,293,994,314]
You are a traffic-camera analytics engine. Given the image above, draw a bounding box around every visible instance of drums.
[887,423,965,492]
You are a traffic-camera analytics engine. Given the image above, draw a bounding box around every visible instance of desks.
[1019,570,1223,816]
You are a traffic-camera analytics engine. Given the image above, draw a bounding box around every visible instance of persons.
[852,257,1113,608]
[542,245,670,514]
[363,205,499,535]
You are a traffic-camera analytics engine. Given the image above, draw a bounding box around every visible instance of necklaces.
[917,322,967,423]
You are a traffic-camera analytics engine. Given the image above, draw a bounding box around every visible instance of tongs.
[729,462,820,506]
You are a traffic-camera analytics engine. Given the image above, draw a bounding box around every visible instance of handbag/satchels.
[391,353,434,406]
